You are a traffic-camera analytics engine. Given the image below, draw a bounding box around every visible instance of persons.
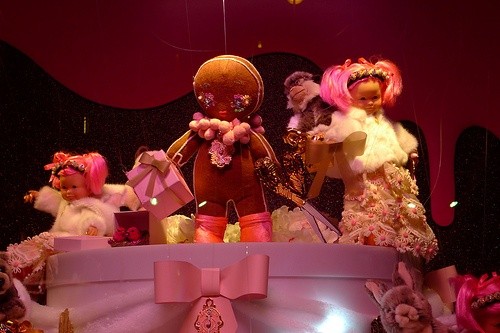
[0,150,117,288]
[166,54,289,244]
[320,60,439,263]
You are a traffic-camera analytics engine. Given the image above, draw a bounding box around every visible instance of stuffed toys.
[364,261,450,333]
